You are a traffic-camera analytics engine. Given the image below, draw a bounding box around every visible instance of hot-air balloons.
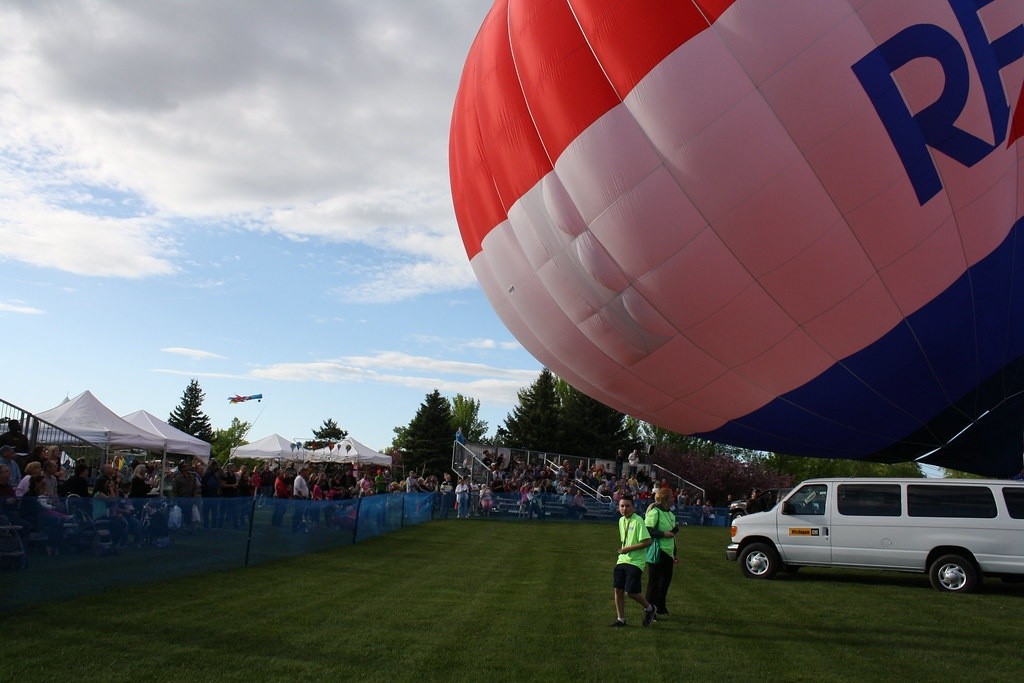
[447,0,1024,493]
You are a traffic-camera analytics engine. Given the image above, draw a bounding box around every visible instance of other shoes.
[465,516,468,519]
[457,515,462,518]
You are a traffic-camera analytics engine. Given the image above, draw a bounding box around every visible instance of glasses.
[3,469,9,473]
[280,473,282,474]
[458,428,461,429]
[247,473,248,475]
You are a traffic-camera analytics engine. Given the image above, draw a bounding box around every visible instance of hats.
[0,445,16,453]
[564,460,568,464]
[377,470,381,473]
[612,476,615,479]
[577,490,581,492]
[547,480,552,484]
[559,481,565,485]
[455,502,457,509]
[8,420,20,426]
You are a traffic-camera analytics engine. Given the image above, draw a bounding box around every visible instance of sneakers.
[657,612,671,616]
[611,620,627,628]
[643,608,658,621]
[642,605,657,627]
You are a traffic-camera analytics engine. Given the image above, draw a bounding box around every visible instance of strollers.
[0,515,29,571]
[66,493,119,558]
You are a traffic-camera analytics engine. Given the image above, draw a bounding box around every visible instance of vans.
[730,488,825,519]
[725,478,1024,591]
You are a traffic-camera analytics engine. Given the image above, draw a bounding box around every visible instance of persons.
[644,487,679,622]
[609,496,657,627]
[0,420,761,571]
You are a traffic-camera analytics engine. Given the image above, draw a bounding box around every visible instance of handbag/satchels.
[646,541,673,568]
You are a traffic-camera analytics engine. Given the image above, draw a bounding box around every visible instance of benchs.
[455,462,694,520]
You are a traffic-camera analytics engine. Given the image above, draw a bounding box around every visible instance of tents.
[0,391,212,499]
[309,436,392,481]
[230,433,312,469]
